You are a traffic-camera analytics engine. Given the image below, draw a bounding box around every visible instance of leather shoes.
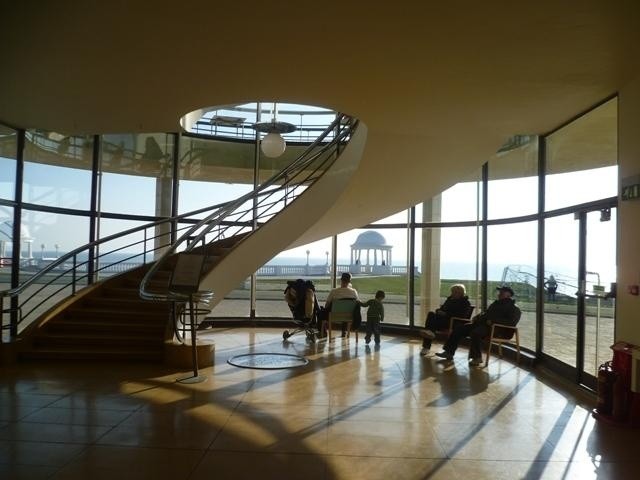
[421,347,482,365]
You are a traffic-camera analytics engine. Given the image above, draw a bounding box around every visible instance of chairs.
[320,298,359,343]
[436,305,521,367]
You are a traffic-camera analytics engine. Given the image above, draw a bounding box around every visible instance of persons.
[419,284,472,355]
[317,273,361,338]
[357,290,385,345]
[436,286,515,365]
[544,275,558,303]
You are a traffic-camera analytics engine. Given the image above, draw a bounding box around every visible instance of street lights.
[326,252,330,275]
[41,243,45,260]
[55,244,62,258]
[306,250,310,276]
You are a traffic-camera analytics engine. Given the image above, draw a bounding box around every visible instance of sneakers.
[420,330,435,339]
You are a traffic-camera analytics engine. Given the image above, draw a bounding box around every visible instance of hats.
[496,286,514,297]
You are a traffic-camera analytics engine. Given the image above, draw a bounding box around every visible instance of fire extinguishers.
[596,361,611,412]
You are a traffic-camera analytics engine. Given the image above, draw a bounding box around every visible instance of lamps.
[250,102,297,158]
